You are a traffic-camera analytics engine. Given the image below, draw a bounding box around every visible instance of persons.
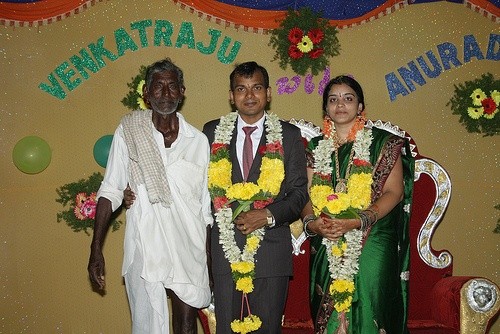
[87,59,214,334]
[300,75,414,334]
[124,62,309,334]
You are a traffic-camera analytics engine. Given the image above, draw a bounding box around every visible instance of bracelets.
[356,209,378,231]
[303,213,317,238]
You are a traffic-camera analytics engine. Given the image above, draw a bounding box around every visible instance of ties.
[242,125,258,182]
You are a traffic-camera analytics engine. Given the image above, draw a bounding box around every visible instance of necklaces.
[331,132,354,193]
[207,109,286,334]
[308,113,375,334]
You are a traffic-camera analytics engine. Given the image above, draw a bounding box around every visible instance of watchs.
[265,208,273,227]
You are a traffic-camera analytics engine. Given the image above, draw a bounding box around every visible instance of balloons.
[12,136,51,174]
[93,135,113,169]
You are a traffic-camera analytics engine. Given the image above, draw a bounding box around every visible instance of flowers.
[445,73,500,137]
[121,64,150,110]
[56,172,123,236]
[267,6,343,75]
[324,192,361,220]
[226,181,269,222]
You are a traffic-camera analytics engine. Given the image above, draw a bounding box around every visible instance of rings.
[243,225,246,230]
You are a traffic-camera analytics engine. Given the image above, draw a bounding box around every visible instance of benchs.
[197,119,500,334]
